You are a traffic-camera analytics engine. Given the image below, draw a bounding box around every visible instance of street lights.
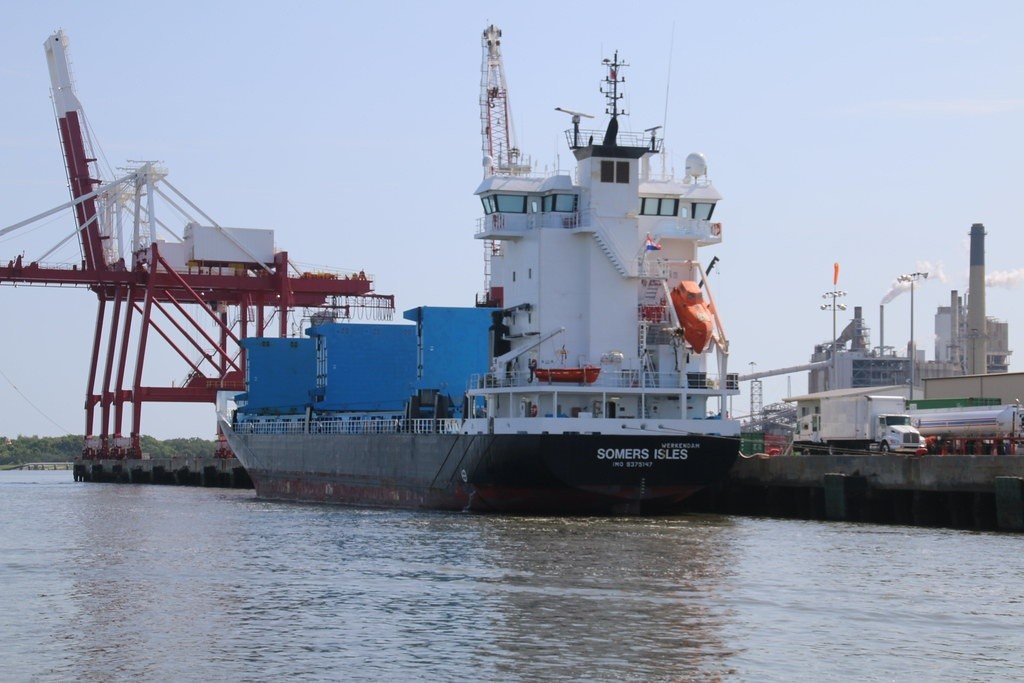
[821,285,847,388]
[897,271,929,400]
[749,361,757,375]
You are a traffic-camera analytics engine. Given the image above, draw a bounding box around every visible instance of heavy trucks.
[819,395,927,455]
[793,402,1024,455]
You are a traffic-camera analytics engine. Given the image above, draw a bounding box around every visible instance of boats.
[671,280,714,354]
[212,22,745,515]
[533,366,602,383]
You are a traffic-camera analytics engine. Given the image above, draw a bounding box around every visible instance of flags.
[646,236,662,250]
